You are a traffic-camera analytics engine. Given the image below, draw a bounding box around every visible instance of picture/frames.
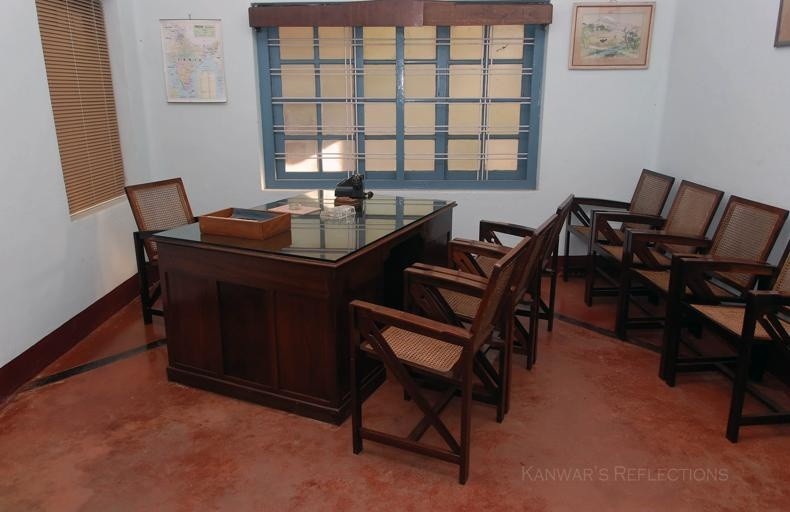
[773,0,790,47]
[568,3,656,69]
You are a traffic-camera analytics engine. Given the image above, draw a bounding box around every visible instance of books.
[268,202,322,216]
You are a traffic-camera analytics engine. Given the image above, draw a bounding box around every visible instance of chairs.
[121,167,789,484]
